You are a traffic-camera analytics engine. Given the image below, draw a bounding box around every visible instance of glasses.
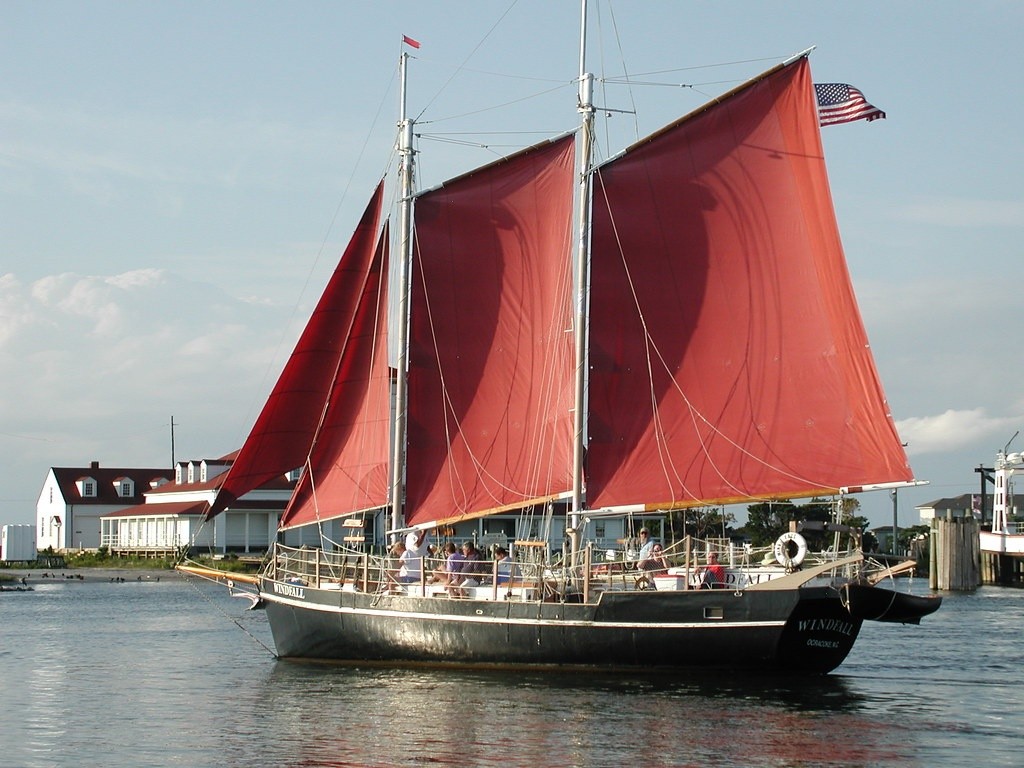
[708,555,715,559]
[641,532,647,535]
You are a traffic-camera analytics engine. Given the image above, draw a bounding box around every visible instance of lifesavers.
[773,531,807,568]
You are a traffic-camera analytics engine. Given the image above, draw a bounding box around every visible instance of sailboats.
[175,0,943,673]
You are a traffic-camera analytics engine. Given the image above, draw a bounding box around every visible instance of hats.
[640,527,649,532]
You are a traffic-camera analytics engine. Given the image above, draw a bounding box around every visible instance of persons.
[493,547,522,584]
[693,552,725,589]
[388,542,420,596]
[406,523,436,557]
[428,541,486,600]
[637,528,671,590]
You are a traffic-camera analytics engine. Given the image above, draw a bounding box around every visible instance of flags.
[401,34,421,49]
[811,82,885,127]
